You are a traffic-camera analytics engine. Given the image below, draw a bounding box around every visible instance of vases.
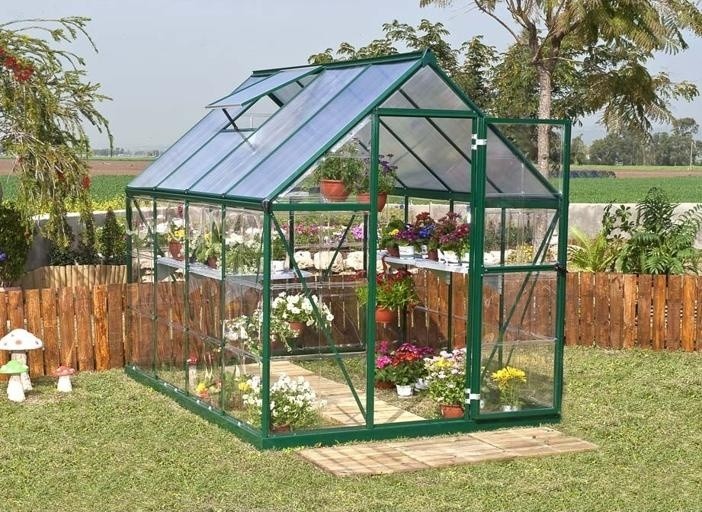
[374,305,404,324]
[270,422,289,434]
[387,245,469,264]
[357,193,387,211]
[169,239,185,260]
[503,405,522,412]
[441,404,465,417]
[376,377,430,397]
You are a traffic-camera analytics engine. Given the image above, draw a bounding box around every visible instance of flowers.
[221,291,335,368]
[239,374,329,431]
[355,152,398,193]
[195,370,252,410]
[424,346,466,404]
[286,223,362,244]
[488,365,529,407]
[375,337,433,385]
[165,218,194,244]
[377,212,469,254]
[354,268,419,315]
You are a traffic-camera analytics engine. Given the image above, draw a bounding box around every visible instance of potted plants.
[300,136,365,200]
[189,220,286,276]
